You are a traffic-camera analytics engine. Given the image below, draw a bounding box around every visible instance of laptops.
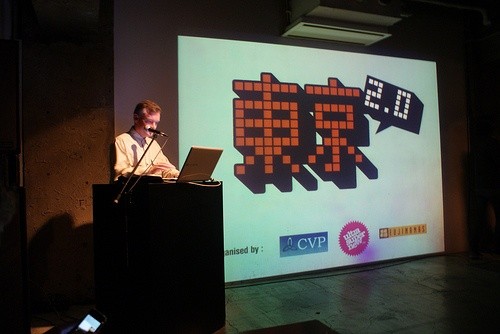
[162,147,223,182]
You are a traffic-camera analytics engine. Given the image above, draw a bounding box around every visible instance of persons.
[106,98,182,195]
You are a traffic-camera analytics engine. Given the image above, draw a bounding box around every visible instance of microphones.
[145,125,168,138]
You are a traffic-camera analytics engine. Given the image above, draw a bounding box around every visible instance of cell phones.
[72,308,107,334]
